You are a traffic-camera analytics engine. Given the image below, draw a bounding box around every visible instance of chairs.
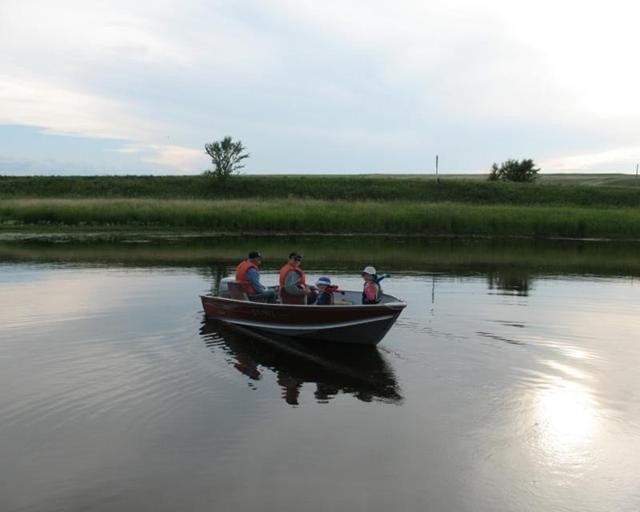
[227,279,308,304]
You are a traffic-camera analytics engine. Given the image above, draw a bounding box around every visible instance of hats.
[289,252,302,258]
[362,266,376,277]
[250,251,261,258]
[316,277,330,286]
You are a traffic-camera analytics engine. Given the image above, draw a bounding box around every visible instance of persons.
[280,253,311,304]
[314,278,334,304]
[362,266,381,304]
[236,251,276,303]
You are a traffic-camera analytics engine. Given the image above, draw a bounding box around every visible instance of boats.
[200,291,407,345]
